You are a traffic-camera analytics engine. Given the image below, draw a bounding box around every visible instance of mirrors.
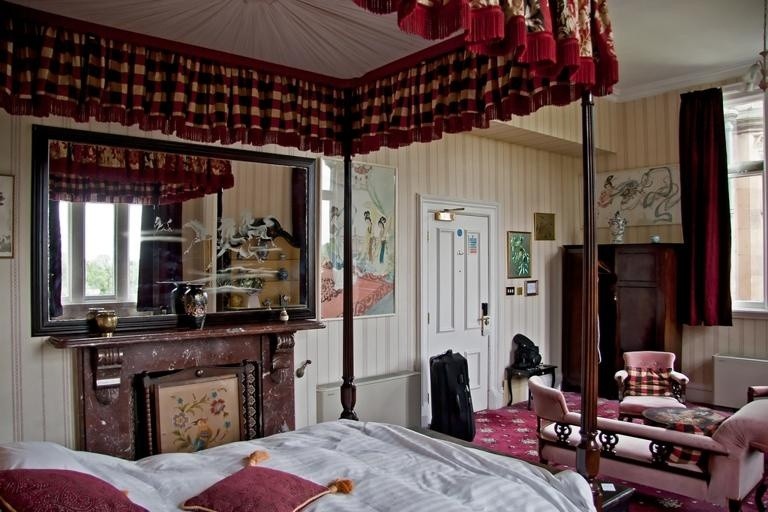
[24,114,329,343]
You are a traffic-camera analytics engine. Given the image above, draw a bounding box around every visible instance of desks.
[634,400,737,477]
[505,364,562,414]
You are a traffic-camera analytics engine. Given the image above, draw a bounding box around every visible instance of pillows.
[667,417,730,464]
[171,446,355,511]
[626,363,672,396]
[2,463,151,512]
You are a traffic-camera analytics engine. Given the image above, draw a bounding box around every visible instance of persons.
[376,217,387,260]
[329,205,341,260]
[361,210,375,260]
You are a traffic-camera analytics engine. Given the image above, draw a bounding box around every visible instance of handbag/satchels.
[514,334,541,370]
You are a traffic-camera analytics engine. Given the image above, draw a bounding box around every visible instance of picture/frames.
[321,146,398,322]
[577,163,681,230]
[0,169,17,261]
[504,208,559,298]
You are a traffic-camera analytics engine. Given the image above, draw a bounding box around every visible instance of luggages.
[430,353,476,443]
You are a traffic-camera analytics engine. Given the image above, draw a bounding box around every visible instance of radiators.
[316,371,423,428]
[710,352,768,410]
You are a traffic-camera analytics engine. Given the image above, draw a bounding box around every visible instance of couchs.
[613,344,692,420]
[529,369,767,511]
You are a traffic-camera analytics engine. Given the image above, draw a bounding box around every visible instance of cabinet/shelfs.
[557,238,686,407]
[219,213,305,309]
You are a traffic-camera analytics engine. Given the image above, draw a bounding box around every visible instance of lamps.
[739,52,766,98]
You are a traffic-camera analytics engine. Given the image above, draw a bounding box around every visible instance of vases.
[182,280,209,330]
[166,275,191,330]
[606,209,628,243]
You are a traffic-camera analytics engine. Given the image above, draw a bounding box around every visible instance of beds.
[3,409,596,511]
